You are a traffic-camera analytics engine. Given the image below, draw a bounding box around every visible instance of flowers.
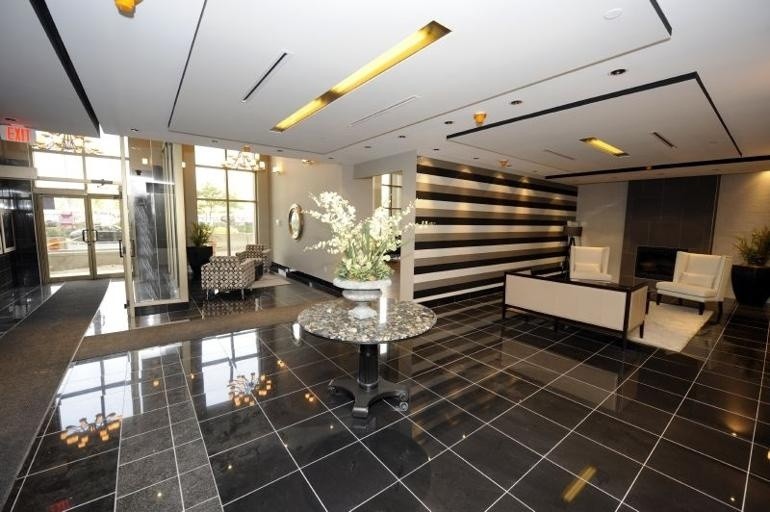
[299,189,440,281]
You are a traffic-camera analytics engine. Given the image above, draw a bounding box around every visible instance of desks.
[296,300,439,418]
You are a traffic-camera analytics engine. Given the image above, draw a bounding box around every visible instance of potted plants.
[729,226,770,307]
[187,219,217,276]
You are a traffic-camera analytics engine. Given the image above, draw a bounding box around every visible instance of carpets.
[0,278,112,511]
[245,272,291,289]
[72,295,337,362]
[610,299,716,354]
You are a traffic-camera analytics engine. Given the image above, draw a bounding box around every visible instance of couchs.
[499,266,649,351]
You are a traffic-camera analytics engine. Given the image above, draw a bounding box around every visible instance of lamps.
[57,411,122,451]
[223,367,273,407]
[32,132,102,158]
[561,224,584,272]
[220,145,268,173]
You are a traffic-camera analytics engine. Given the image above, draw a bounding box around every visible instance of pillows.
[575,263,601,272]
[678,271,715,288]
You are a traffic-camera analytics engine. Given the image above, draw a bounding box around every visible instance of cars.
[69,225,122,243]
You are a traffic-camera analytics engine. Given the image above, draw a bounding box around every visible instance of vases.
[332,276,392,321]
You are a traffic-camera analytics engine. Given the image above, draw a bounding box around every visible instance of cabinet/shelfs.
[0,158,46,317]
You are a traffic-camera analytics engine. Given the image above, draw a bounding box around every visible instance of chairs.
[567,246,614,289]
[654,249,728,316]
[200,243,271,301]
